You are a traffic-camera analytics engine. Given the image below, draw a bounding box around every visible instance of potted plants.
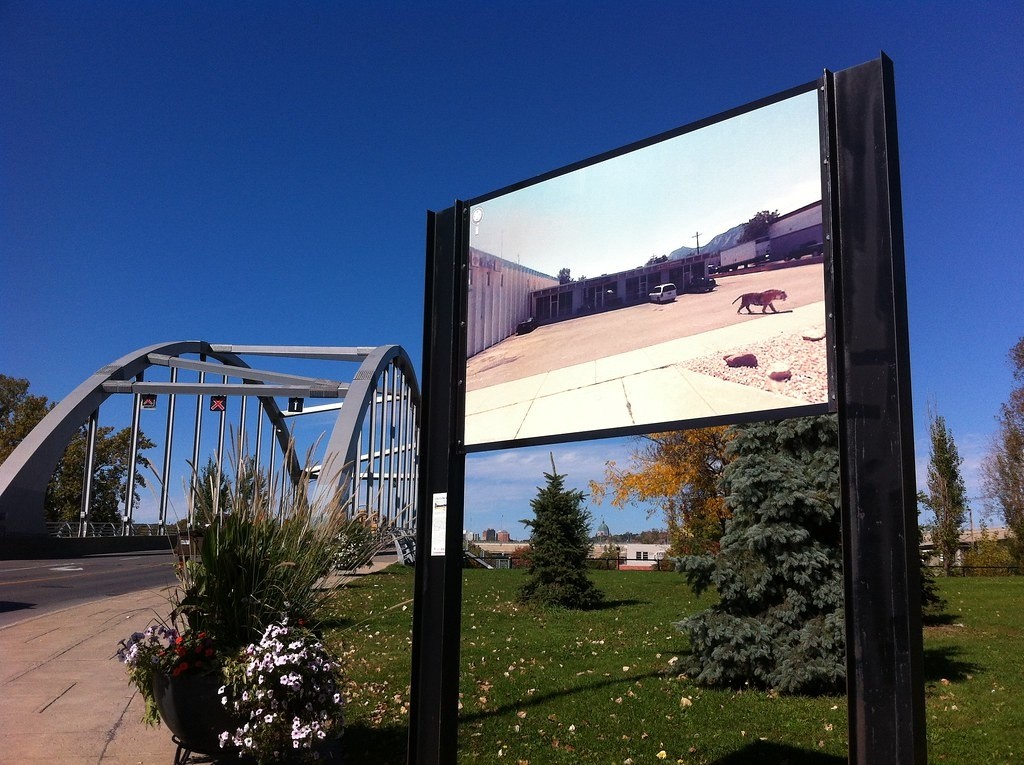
[328,523,375,571]
[131,420,420,762]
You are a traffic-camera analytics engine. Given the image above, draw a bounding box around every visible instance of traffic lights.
[209,395,228,412]
[287,398,305,413]
[140,393,158,410]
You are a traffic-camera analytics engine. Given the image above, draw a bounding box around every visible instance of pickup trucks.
[783,240,823,262]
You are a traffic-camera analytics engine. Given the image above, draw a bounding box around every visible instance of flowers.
[324,533,370,568]
[111,561,351,760]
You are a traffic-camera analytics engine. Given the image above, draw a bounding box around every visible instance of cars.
[684,278,717,293]
[516,317,538,335]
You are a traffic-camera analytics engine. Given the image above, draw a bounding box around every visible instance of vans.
[647,283,678,304]
